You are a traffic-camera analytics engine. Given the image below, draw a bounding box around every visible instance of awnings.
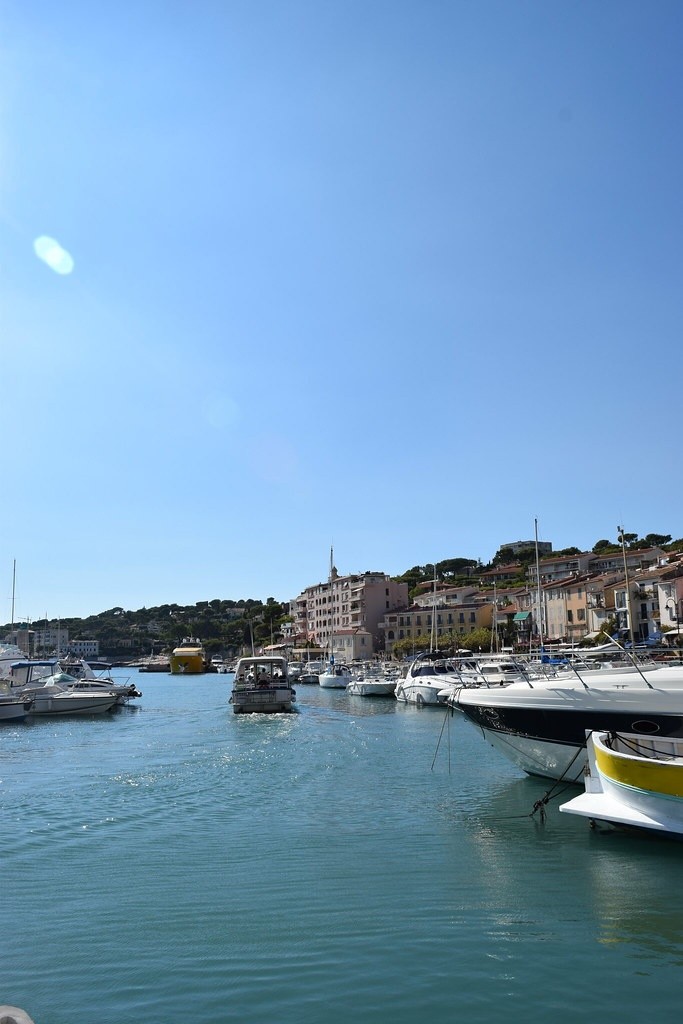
[512,611,531,621]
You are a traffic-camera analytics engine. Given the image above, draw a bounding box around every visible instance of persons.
[246,668,279,685]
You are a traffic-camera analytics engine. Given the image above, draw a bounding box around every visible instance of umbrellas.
[611,631,665,649]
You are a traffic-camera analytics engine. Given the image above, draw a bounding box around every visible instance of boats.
[287,519,669,708]
[0,559,143,715]
[168,632,206,674]
[434,648,683,784]
[211,654,223,666]
[228,656,297,714]
[558,729,683,834]
[0,676,36,721]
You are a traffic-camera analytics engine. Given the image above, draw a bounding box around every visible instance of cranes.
[18,616,31,629]
